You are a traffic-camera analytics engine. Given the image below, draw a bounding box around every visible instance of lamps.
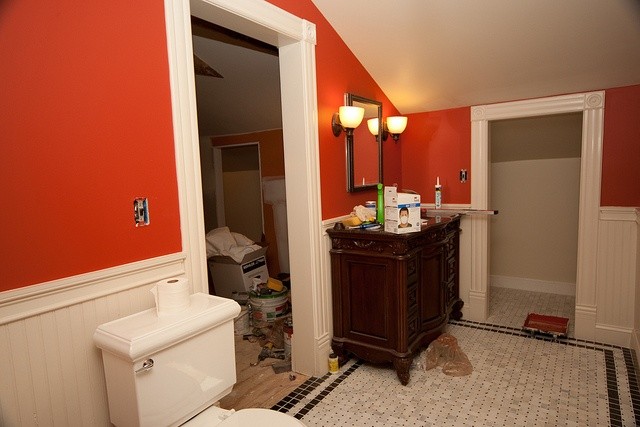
[333,102,366,135]
[366,117,379,139]
[387,114,408,139]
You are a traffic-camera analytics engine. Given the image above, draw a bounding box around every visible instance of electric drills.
[254,342,284,366]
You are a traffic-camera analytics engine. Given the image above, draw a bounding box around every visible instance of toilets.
[94,294,307,427]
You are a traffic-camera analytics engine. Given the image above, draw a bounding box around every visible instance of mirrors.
[345,94,384,192]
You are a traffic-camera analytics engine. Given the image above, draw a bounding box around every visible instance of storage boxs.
[208,247,270,297]
[382,186,421,233]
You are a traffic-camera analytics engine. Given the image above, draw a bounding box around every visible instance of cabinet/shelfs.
[325,216,462,385]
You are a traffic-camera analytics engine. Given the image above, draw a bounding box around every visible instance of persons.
[397,208,411,228]
